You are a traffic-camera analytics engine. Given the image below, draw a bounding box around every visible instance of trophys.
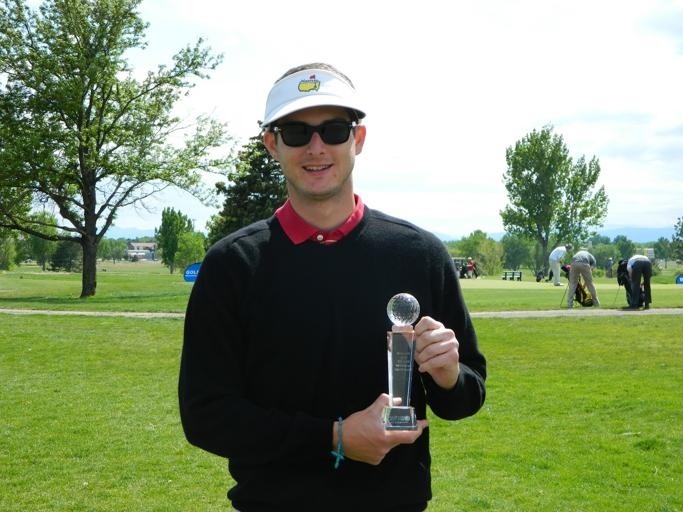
[375,293,421,430]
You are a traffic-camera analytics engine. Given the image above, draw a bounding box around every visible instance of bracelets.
[331,417,344,469]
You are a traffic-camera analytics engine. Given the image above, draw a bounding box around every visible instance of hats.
[260,69,366,127]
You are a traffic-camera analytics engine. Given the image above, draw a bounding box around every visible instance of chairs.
[502,271,522,282]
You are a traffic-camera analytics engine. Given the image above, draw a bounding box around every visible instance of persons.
[549,243,652,310]
[464,257,474,278]
[179,63,486,508]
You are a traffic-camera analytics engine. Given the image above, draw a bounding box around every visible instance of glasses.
[270,120,352,146]
